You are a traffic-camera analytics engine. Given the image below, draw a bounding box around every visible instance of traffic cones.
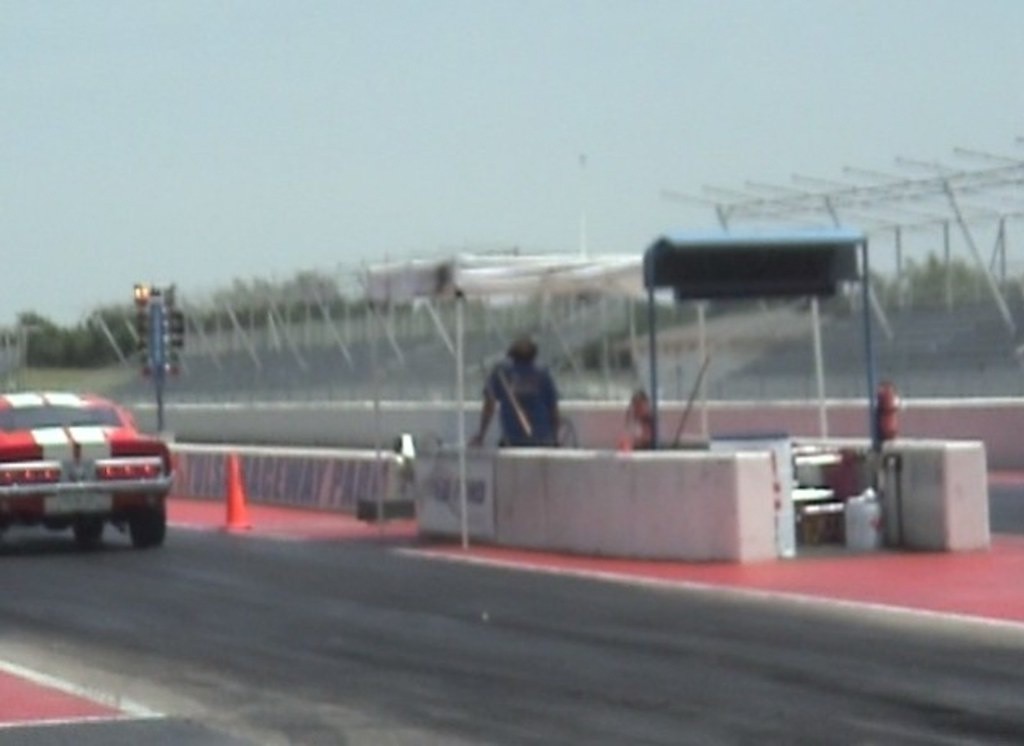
[218,453,256,531]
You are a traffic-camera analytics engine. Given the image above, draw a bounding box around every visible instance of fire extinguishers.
[625,387,652,448]
[876,380,900,440]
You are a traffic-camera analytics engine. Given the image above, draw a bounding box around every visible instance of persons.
[471,336,560,446]
[620,392,660,451]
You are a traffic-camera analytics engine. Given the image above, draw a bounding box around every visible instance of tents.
[362,253,830,549]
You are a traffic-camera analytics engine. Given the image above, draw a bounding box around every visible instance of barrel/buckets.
[843,495,886,552]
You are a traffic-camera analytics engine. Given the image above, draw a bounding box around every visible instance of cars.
[0,389,177,547]
